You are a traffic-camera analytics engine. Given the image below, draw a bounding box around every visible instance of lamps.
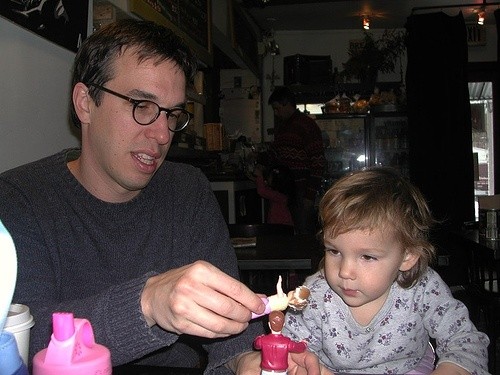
[478,10,485,26]
[361,14,373,31]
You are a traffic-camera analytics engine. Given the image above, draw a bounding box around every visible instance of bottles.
[32,311,112,375]
[0,332,31,375]
[376,128,408,176]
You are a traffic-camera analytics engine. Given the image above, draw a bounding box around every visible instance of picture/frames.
[228,0,264,81]
[127,0,215,68]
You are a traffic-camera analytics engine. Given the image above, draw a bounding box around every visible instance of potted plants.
[331,22,406,83]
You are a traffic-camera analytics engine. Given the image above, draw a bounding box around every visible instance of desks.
[228,224,312,270]
[441,222,500,375]
[207,180,266,225]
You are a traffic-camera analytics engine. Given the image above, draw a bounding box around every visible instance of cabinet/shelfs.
[369,103,410,184]
[308,113,369,214]
[93,0,212,161]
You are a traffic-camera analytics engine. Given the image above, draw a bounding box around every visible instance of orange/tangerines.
[351,100,367,109]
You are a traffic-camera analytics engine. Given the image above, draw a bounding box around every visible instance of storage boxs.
[283,53,332,86]
[203,122,225,151]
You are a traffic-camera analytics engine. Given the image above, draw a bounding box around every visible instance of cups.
[3,303,36,369]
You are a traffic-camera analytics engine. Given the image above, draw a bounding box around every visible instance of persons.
[253,311,308,375]
[251,275,311,319]
[0,20,334,375]
[254,91,326,260]
[281,166,490,375]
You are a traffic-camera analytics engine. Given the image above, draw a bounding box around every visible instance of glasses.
[83,81,193,133]
